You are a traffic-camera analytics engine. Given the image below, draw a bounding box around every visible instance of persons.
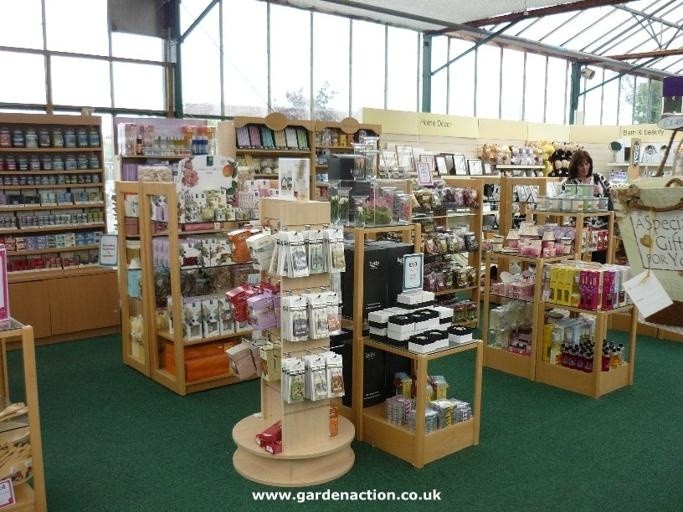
[560,150,615,264]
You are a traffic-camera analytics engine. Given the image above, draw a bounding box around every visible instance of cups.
[352,134,380,179]
[324,180,415,225]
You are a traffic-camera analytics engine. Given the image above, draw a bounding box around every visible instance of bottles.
[0,125,100,171]
[560,338,626,375]
[124,131,210,182]
[530,183,609,213]
[437,297,479,322]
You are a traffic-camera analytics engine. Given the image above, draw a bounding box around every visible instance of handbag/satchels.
[593,173,618,222]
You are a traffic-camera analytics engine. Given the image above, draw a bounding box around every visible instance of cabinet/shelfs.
[0,106,682,512]
